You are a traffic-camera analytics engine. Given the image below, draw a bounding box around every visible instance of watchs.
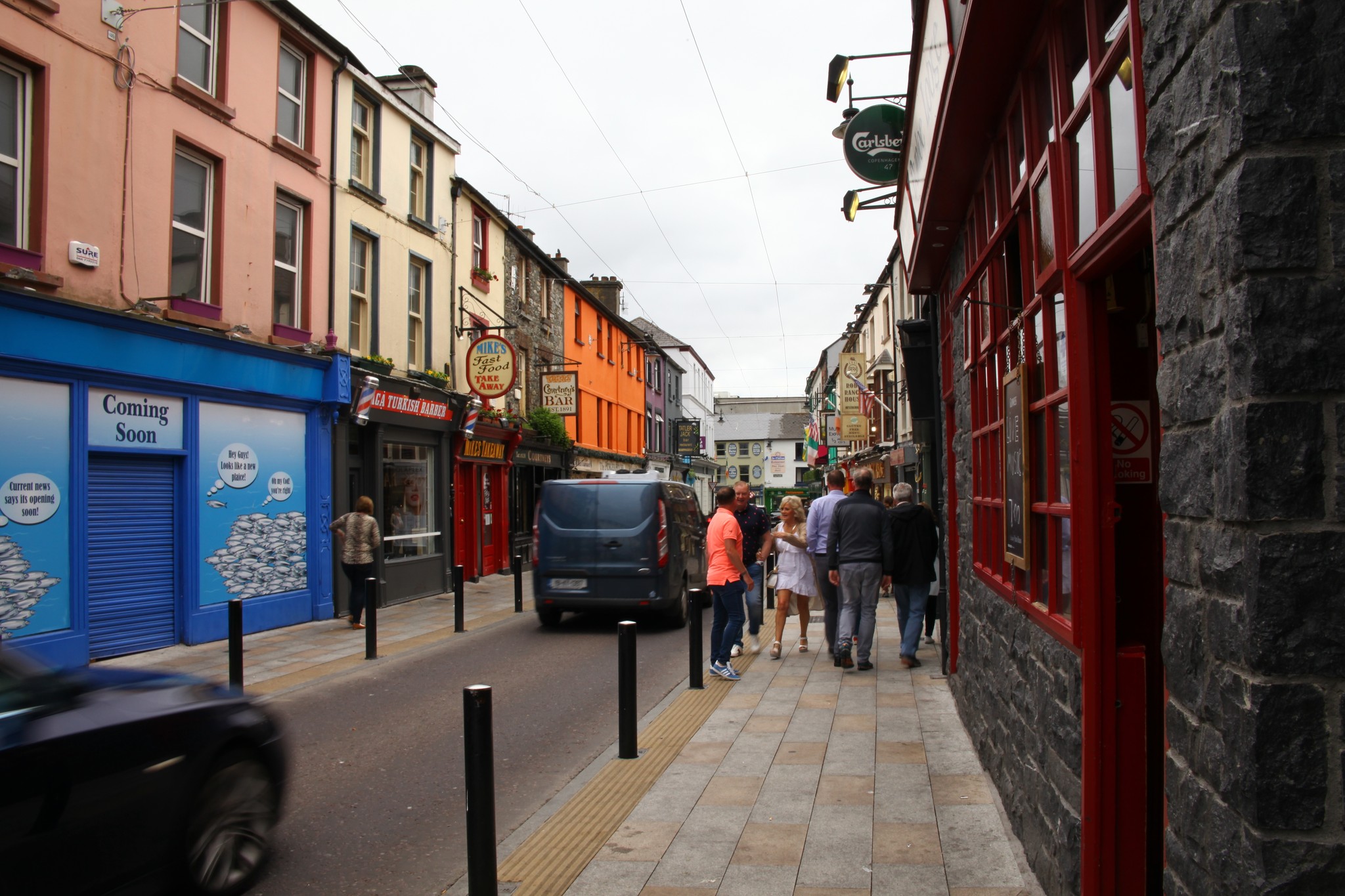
[756,560,764,566]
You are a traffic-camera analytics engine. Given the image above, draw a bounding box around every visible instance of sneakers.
[925,636,935,644]
[709,660,741,680]
[749,635,761,654]
[730,644,743,657]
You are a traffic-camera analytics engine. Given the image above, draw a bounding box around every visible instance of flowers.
[474,264,499,282]
[363,354,395,368]
[425,369,451,382]
[481,406,518,419]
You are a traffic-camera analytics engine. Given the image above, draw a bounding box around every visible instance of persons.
[916,501,940,643]
[845,491,862,644]
[803,499,815,518]
[804,469,850,668]
[390,471,429,558]
[706,486,756,681]
[708,506,719,526]
[329,496,380,630]
[824,466,895,670]
[730,481,773,657]
[756,495,825,659]
[881,483,940,667]
[883,494,895,598]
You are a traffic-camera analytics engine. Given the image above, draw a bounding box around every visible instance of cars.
[0,638,287,896]
[770,510,782,526]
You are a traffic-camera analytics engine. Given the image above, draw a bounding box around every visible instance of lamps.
[620,340,661,369]
[467,330,475,343]
[766,441,772,447]
[698,453,715,463]
[455,326,464,341]
[706,408,725,426]
[840,282,891,340]
[827,49,910,223]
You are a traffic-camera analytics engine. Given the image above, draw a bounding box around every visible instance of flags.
[854,378,876,419]
[802,425,809,462]
[807,413,819,467]
[834,407,842,434]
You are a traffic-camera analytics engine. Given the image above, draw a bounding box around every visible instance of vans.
[529,469,716,628]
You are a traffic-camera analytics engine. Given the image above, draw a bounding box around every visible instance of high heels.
[770,641,782,657]
[799,636,808,652]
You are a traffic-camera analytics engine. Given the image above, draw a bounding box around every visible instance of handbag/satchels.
[765,566,779,589]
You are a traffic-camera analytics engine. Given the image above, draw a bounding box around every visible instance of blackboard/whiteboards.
[1002,364,1031,571]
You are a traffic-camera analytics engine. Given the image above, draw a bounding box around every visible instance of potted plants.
[522,407,577,450]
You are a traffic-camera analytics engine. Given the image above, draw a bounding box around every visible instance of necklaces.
[786,522,796,526]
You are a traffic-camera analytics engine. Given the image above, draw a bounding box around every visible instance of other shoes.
[828,648,840,666]
[899,653,921,668]
[857,661,873,670]
[348,616,365,630]
[840,646,854,668]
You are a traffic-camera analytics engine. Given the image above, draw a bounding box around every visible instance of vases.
[467,410,492,424]
[407,369,449,388]
[473,271,489,286]
[492,417,514,428]
[356,358,393,376]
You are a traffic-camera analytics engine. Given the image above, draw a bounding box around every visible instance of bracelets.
[740,569,748,576]
